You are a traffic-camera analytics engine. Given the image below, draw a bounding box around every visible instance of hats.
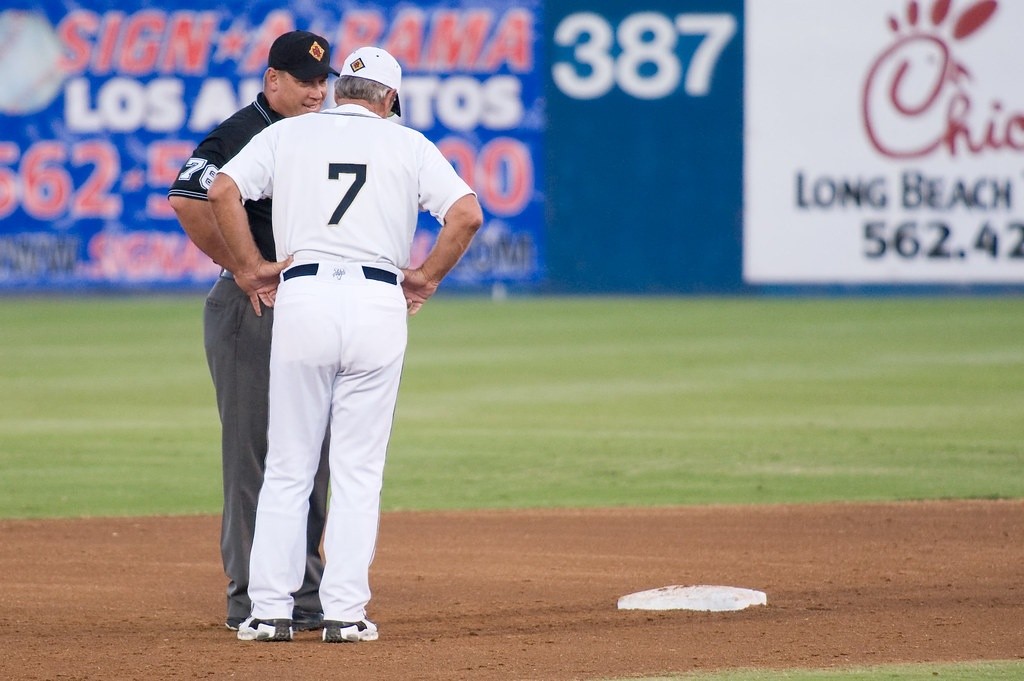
[340,47,402,118]
[268,29,341,80]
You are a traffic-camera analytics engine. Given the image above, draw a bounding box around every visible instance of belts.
[219,265,234,280]
[283,263,398,287]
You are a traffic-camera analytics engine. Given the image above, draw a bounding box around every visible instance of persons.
[208,45,483,642]
[167,31,341,631]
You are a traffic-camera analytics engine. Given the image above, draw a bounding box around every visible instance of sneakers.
[225,615,246,631]
[322,619,379,642]
[290,609,323,632]
[237,616,293,641]
